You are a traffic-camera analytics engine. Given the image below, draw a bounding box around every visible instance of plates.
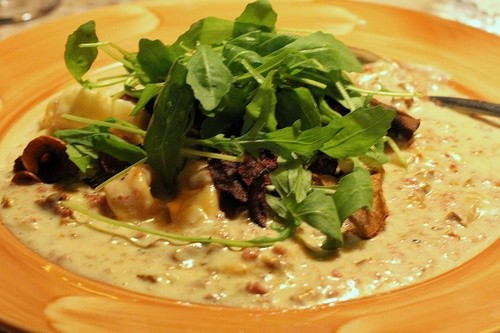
[0,0,500,333]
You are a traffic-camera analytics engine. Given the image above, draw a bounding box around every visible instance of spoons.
[345,44,500,119]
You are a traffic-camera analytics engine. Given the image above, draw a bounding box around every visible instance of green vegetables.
[50,0,421,249]
